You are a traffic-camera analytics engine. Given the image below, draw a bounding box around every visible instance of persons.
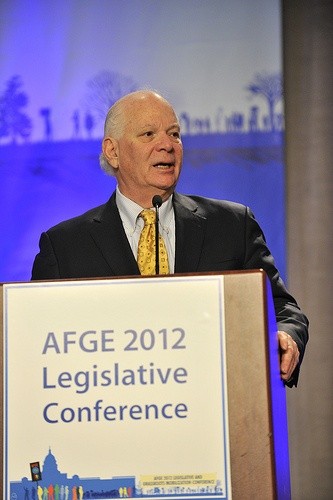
[27,88,310,390]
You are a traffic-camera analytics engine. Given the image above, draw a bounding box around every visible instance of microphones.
[152,195,162,275]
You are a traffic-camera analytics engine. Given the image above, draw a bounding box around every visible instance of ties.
[136,209,169,275]
